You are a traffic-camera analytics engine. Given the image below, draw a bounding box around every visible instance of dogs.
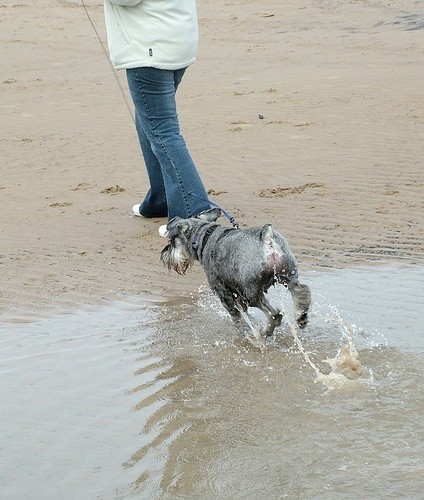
[158,207,310,338]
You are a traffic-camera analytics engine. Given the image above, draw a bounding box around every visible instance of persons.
[100,0,217,238]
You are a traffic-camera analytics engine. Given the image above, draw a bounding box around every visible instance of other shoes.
[158,223,172,237]
[132,202,144,218]
